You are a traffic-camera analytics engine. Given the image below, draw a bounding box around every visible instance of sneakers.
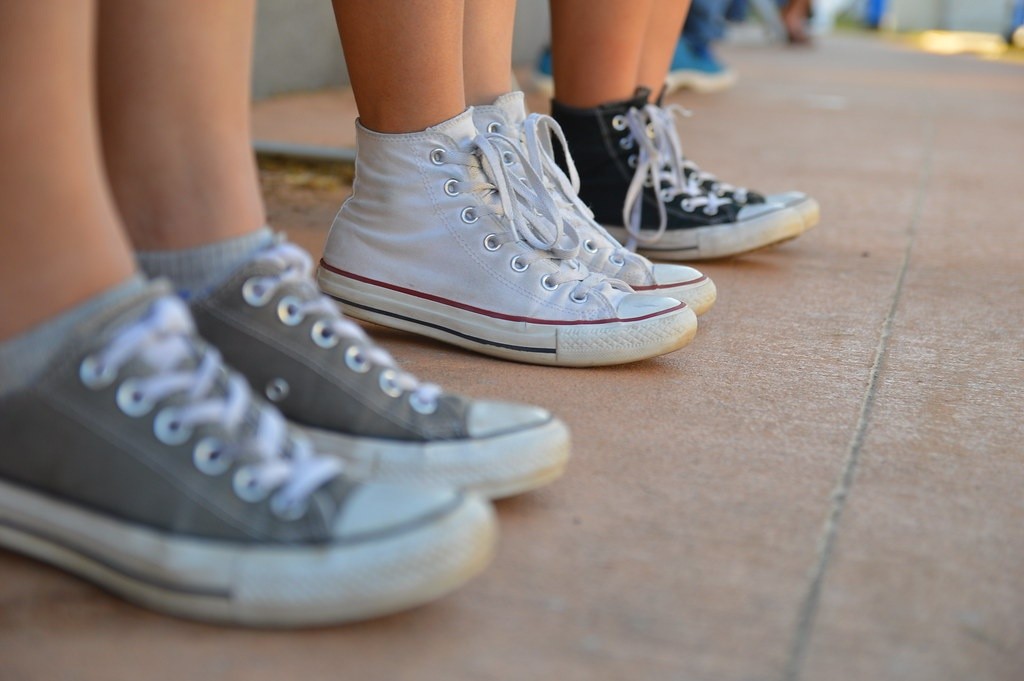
[0,278,498,628]
[314,89,718,367]
[187,229,571,502]
[547,83,820,262]
[665,35,734,92]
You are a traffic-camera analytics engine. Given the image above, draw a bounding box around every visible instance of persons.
[547,1,822,263]
[310,1,719,370]
[1,0,574,629]
[531,2,816,98]
[1007,4,1023,55]
